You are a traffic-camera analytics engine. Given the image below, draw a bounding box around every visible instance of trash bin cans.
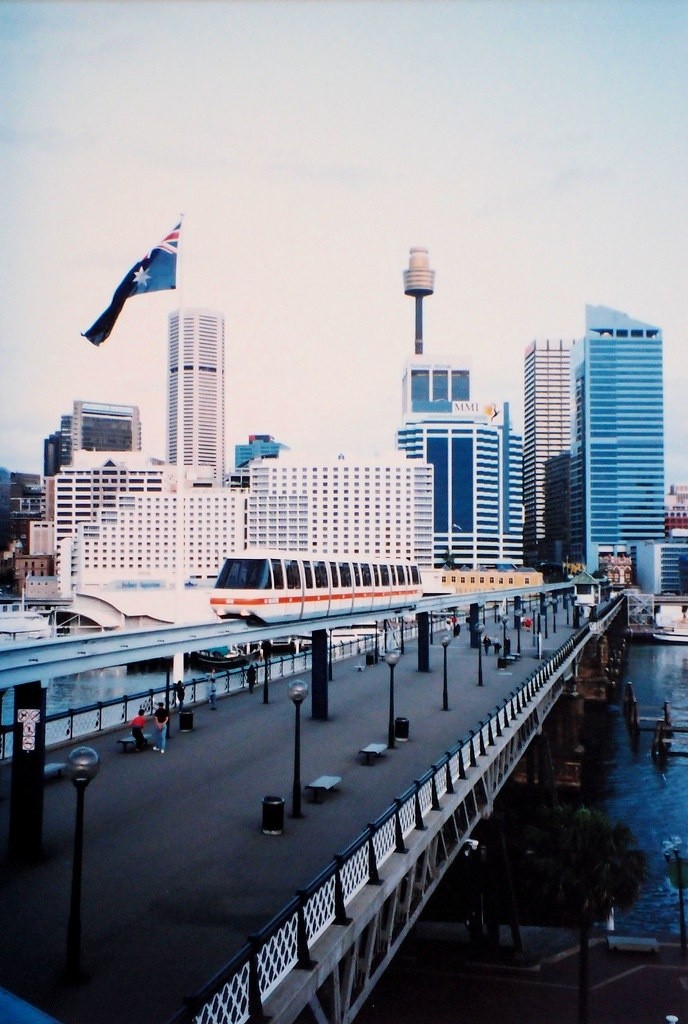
[179,712,194,732]
[395,717,409,741]
[499,659,506,668]
[366,654,374,665]
[261,795,285,836]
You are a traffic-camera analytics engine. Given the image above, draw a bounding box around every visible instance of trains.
[210,548,424,627]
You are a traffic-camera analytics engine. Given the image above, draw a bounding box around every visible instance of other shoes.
[161,748,164,754]
[153,746,160,751]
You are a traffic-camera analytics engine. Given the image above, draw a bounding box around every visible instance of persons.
[246,665,256,693]
[129,709,147,751]
[483,635,501,655]
[177,681,185,713]
[446,616,457,630]
[671,606,688,629]
[522,618,532,632]
[209,678,217,709]
[153,703,168,753]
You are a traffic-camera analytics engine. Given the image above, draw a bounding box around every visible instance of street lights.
[65,746,100,964]
[287,679,309,818]
[440,634,451,711]
[385,651,401,748]
[475,623,485,687]
[501,596,570,658]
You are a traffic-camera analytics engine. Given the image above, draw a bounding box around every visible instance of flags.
[80,222,181,346]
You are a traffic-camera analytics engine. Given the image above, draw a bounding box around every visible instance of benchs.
[116,734,153,752]
[358,743,387,767]
[44,762,67,778]
[303,775,341,804]
[505,653,521,663]
